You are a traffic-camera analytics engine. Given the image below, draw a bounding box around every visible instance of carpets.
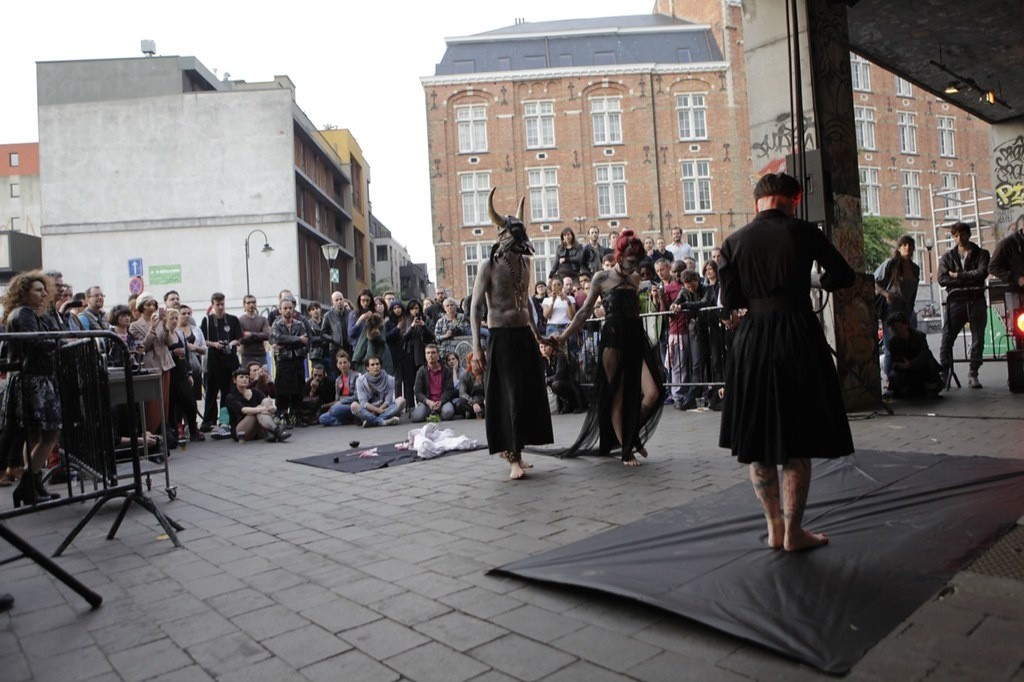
[284,438,486,475]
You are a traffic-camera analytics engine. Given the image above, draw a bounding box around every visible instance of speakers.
[1006,351,1024,394]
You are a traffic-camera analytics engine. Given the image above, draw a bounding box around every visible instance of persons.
[873,235,920,394]
[883,311,945,394]
[987,214,1024,308]
[350,352,407,428]
[717,171,857,552]
[223,367,291,444]
[522,229,669,468]
[201,225,741,445]
[0,267,206,511]
[937,221,990,389]
[410,342,455,422]
[469,186,557,481]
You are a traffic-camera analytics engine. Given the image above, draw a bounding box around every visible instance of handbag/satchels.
[190,352,202,377]
[642,294,662,347]
[874,292,887,318]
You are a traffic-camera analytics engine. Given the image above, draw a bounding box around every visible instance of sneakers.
[210,427,231,440]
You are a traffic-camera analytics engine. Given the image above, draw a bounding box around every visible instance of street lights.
[923,238,934,302]
[245,229,275,295]
[321,243,340,294]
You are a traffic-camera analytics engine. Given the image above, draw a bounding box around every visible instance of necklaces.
[501,254,526,313]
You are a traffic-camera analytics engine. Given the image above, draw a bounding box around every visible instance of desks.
[109,373,178,500]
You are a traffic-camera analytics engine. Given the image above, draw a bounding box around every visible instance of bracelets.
[149,326,156,333]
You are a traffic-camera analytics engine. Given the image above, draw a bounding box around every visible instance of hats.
[135,293,156,310]
[754,172,804,199]
[281,295,296,302]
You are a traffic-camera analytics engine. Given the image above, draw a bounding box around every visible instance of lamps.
[977,90,996,105]
[944,80,969,94]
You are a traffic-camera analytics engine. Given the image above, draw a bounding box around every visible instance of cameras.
[219,339,232,355]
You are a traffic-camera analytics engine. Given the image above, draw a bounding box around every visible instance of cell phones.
[416,313,422,322]
[158,307,165,319]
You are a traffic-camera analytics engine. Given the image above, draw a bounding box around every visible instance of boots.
[189,422,205,441]
[177,424,186,443]
[13,471,61,507]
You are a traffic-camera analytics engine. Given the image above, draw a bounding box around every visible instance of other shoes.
[405,406,415,412]
[383,416,400,426]
[883,373,889,389]
[664,396,698,410]
[295,422,308,427]
[967,370,982,389]
[265,428,292,442]
[559,406,571,414]
[0,467,27,487]
[363,420,371,427]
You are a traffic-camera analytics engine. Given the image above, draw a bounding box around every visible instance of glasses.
[55,283,66,286]
[361,298,371,302]
[88,293,104,297]
[245,301,256,305]
[436,292,445,295]
